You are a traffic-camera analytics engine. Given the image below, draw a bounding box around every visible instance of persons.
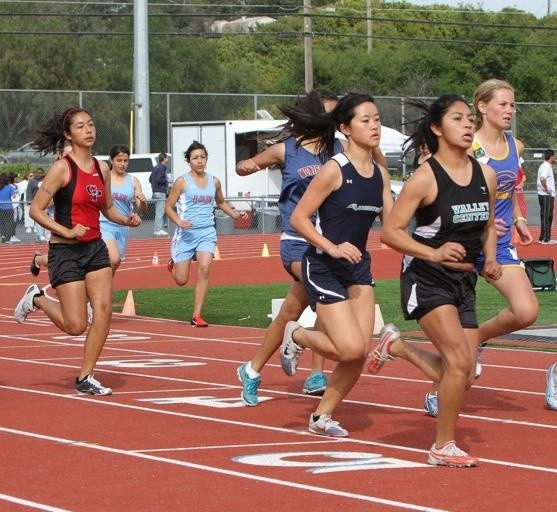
[0,168,55,277]
[86,144,147,325]
[149,152,169,236]
[280,94,395,436]
[516,165,526,218]
[425,81,539,416]
[368,96,504,467]
[164,140,249,327]
[14,108,141,396]
[236,91,388,406]
[536,149,557,245]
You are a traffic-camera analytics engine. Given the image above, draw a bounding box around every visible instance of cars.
[0,142,59,164]
[337,124,415,174]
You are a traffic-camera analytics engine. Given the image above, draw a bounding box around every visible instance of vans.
[91,154,173,220]
[170,110,405,227]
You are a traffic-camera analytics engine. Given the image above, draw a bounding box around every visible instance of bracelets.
[513,217,528,224]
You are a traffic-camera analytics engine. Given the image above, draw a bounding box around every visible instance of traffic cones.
[261,243,269,257]
[149,251,161,270]
[212,244,221,261]
[121,290,137,317]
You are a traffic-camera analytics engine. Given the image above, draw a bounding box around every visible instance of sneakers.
[235,364,261,407]
[475,343,487,378]
[426,438,479,467]
[35,235,49,242]
[26,228,32,234]
[31,253,42,277]
[302,375,328,394]
[542,240,557,244]
[544,362,557,410]
[87,302,94,326]
[154,230,167,235]
[279,320,306,377]
[308,412,350,437]
[365,323,402,375]
[10,236,21,242]
[75,373,113,395]
[423,393,438,417]
[190,314,208,327]
[14,284,44,324]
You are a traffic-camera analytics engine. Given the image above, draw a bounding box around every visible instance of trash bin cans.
[215,210,234,234]
[256,206,280,233]
[167,206,178,238]
